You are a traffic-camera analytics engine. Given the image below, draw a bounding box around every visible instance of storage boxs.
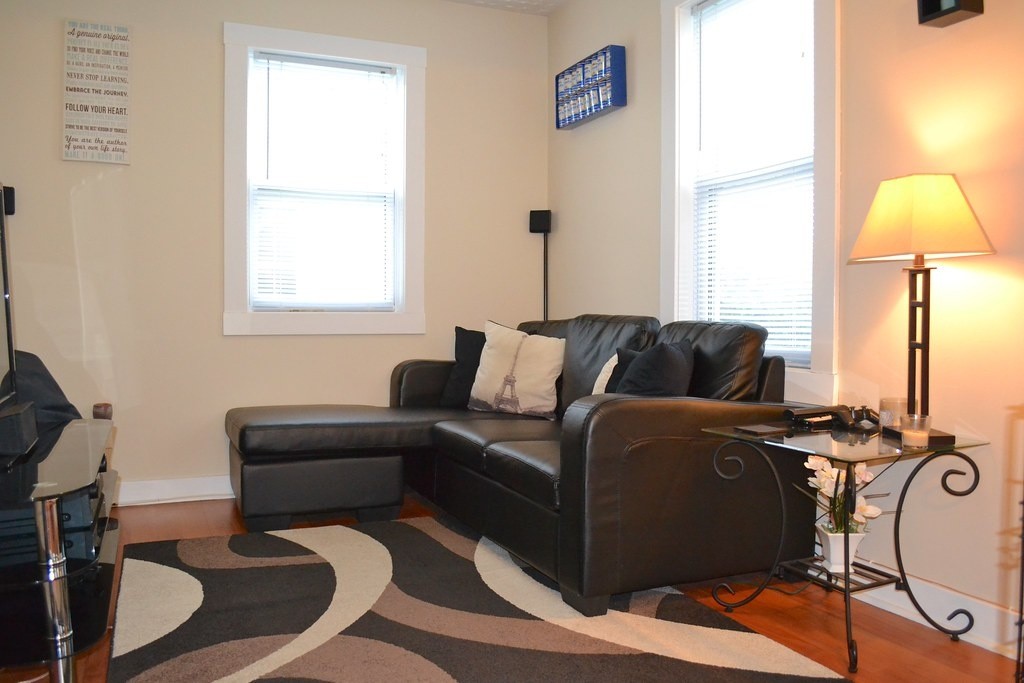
[0,400,40,458]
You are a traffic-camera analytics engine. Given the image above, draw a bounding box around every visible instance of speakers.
[3,186,16,215]
[530,210,551,233]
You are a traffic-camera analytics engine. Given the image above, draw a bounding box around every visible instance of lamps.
[846,172,997,447]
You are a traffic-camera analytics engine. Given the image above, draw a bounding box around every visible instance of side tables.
[699,422,991,673]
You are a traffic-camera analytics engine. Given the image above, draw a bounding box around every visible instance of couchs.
[226,314,818,616]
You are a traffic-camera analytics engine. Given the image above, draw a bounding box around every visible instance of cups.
[900,414,932,452]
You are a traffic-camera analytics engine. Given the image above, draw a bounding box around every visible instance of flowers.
[792,454,882,533]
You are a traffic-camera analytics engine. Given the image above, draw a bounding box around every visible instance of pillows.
[467,320,567,422]
[440,325,486,411]
[592,347,643,394]
[615,338,694,395]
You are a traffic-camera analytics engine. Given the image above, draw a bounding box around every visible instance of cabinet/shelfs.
[555,45,627,130]
[0,419,125,683]
[917,0,984,29]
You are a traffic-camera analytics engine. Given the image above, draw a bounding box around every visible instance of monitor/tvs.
[0,189,18,411]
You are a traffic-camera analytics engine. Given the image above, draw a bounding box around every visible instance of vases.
[814,523,865,575]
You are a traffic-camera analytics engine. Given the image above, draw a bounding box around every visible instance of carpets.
[106,516,860,682]
